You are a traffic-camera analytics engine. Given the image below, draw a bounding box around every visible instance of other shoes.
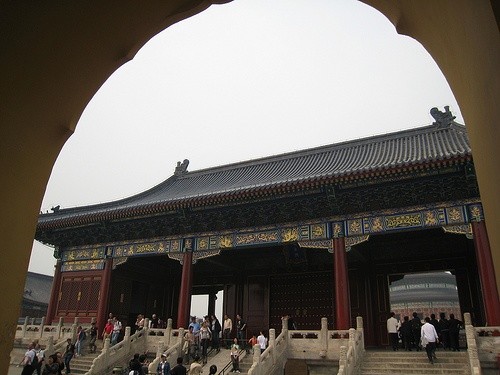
[233,370,236,373]
[236,370,240,373]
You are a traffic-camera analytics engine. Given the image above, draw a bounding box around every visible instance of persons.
[384,312,463,353]
[17,311,297,375]
[420,317,441,364]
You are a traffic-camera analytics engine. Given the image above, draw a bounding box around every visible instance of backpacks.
[30,352,38,365]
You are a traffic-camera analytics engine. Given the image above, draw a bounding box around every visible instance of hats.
[161,354,167,358]
